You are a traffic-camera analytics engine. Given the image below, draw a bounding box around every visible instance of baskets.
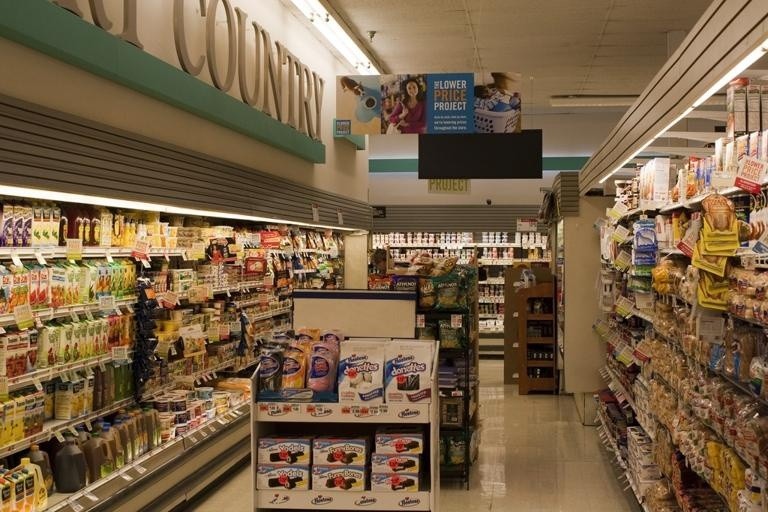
[473,107,520,133]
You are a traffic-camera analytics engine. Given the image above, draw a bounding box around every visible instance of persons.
[381,73,426,134]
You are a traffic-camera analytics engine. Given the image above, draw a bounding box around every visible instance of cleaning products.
[257,323,342,391]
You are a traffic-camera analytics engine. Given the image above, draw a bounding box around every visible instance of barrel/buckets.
[19,405,162,512]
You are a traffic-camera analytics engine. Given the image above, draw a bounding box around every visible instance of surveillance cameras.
[487,199,491,204]
